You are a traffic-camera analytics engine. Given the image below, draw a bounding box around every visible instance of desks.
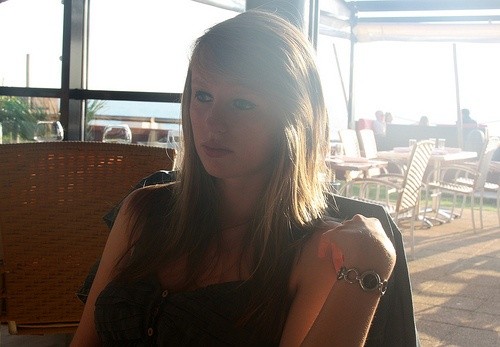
[324,155,389,199]
[376,149,478,226]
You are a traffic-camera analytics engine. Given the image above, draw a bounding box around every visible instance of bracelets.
[337,265,388,296]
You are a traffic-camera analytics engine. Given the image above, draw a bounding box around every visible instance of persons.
[419,116,428,126]
[375,111,392,124]
[67,11,397,345]
[456,109,476,126]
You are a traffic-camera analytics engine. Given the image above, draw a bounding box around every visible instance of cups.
[102,124,132,145]
[438,138,446,150]
[409,139,416,153]
[34,121,64,144]
[430,138,436,148]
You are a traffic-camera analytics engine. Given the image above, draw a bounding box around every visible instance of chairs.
[0,128,500,347]
